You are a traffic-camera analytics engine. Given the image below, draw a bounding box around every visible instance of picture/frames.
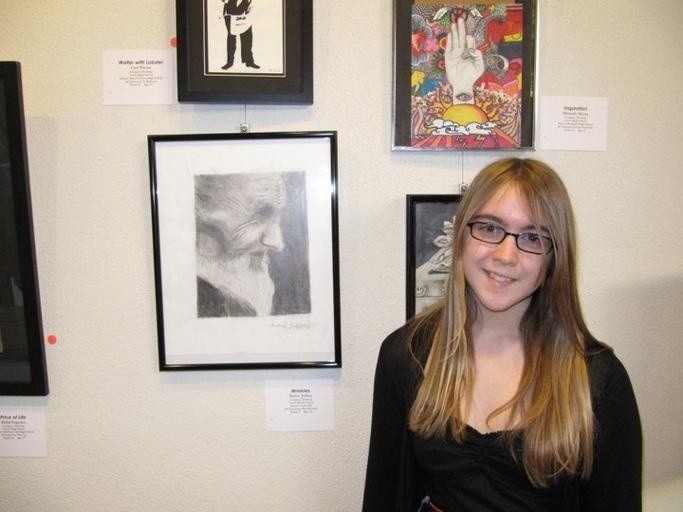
[172,0,314,108]
[392,0,538,153]
[405,194,464,325]
[144,130,344,374]
[0,61,50,398]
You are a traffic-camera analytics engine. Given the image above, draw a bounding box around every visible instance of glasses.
[466,221,553,256]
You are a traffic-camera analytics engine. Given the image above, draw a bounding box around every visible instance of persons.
[444,16,485,108]
[216,1,260,70]
[195,173,310,316]
[360,157,642,510]
